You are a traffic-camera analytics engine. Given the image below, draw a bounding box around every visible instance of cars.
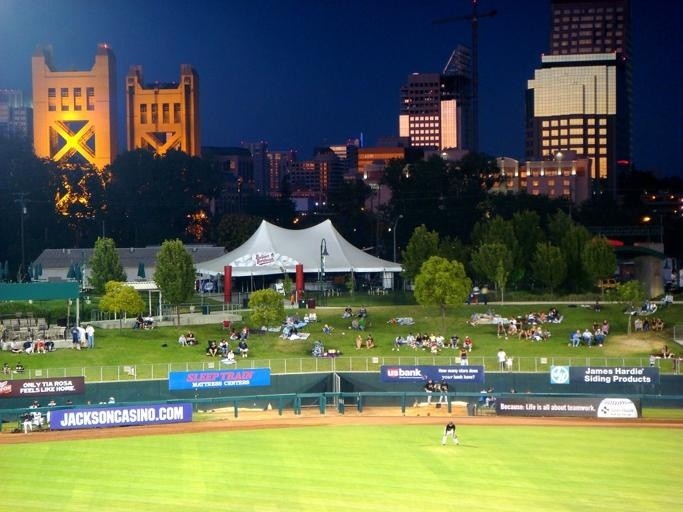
[665,283,681,294]
[373,280,382,290]
[203,282,213,293]
[596,278,621,294]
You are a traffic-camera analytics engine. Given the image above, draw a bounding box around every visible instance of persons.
[134,314,155,330]
[440,420,459,446]
[629,292,674,331]
[470,287,488,305]
[1,362,24,376]
[498,349,512,370]
[1,322,94,354]
[595,301,601,312]
[423,378,448,402]
[178,326,249,361]
[478,388,496,408]
[23,399,57,433]
[282,304,480,357]
[649,344,673,367]
[508,307,609,348]
[459,347,469,366]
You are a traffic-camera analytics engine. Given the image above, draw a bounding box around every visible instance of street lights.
[394,215,404,263]
[76,296,91,327]
[21,205,28,269]
[321,239,329,306]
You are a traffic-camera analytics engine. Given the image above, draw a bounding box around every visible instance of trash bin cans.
[299,298,315,309]
[203,306,210,314]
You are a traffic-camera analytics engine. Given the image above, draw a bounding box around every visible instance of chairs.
[569,331,582,346]
[593,304,596,312]
[582,335,593,344]
[635,320,643,331]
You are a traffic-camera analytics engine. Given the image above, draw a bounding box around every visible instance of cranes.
[431,0,497,154]
